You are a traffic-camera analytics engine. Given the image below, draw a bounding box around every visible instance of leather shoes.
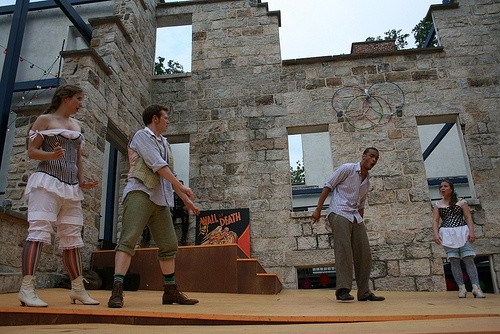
[336,289,354,300]
[162,284,199,305]
[107,282,124,308]
[358,292,385,301]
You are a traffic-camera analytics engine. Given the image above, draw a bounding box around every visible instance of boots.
[17,275,49,307]
[69,275,100,305]
[472,284,486,298]
[458,284,467,298]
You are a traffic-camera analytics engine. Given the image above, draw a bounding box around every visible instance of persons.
[108,103,200,308]
[140,226,152,248]
[169,179,191,245]
[432,179,486,298]
[311,147,387,302]
[16,83,99,306]
